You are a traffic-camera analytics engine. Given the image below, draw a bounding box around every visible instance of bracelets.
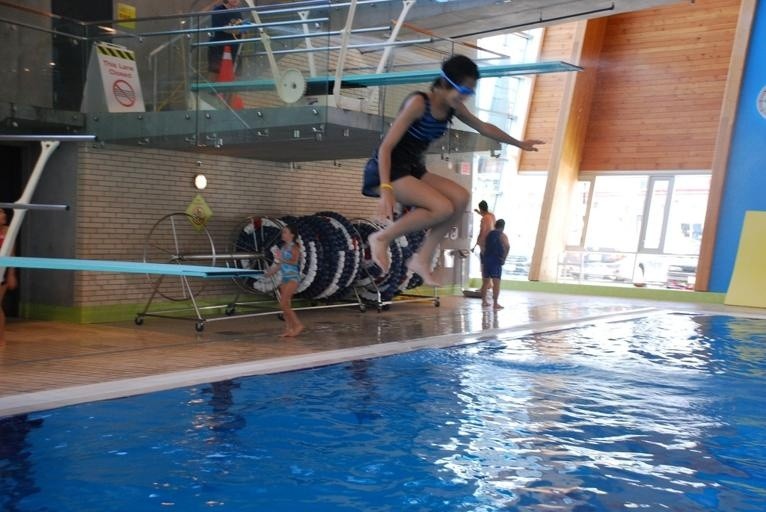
[378,183,394,189]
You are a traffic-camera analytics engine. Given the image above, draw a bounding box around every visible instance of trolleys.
[128,209,363,330]
[350,219,443,312]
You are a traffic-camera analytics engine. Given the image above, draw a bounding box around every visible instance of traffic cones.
[210,43,247,110]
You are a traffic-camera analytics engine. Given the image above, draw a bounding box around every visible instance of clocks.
[756,87,766,119]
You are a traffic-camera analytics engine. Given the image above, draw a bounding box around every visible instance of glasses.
[439,70,475,96]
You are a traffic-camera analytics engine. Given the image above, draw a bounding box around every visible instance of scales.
[450,248,492,298]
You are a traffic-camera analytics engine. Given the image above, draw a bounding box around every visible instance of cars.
[502,247,698,290]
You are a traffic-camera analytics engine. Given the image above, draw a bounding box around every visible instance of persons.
[483,218,510,310]
[471,199,497,293]
[360,54,547,291]
[264,224,305,338]
